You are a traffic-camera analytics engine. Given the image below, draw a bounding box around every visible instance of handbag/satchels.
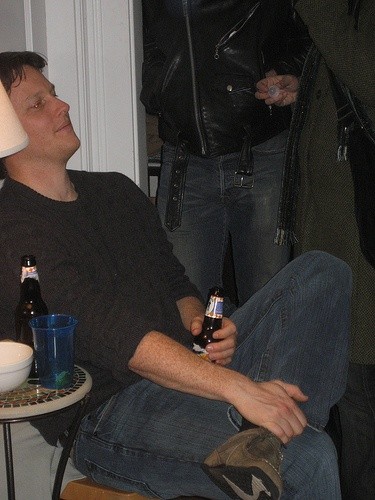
[336,117,375,265]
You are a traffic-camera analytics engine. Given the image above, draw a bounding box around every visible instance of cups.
[29,314,79,389]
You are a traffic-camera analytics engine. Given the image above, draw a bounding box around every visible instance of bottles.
[16,255,49,378]
[191,288,224,364]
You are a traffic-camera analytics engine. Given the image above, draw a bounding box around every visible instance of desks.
[0,365,92,500]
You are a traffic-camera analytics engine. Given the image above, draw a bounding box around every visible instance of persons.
[135,1,313,310]
[256,0,375,498]
[0,49,355,500]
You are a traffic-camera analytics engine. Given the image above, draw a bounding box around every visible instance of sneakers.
[200,425,288,500]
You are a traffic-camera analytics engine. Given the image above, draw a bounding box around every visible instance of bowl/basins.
[0,341,33,393]
